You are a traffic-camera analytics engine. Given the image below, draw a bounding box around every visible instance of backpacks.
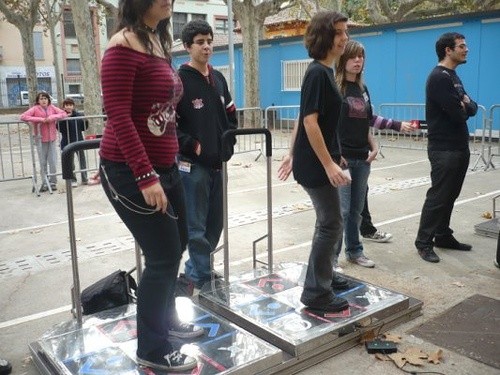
[79,268,137,313]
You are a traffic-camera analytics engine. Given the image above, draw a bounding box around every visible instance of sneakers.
[168,320,204,338]
[347,255,375,267]
[362,230,393,242]
[136,349,197,372]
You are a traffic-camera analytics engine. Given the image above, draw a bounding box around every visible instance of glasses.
[452,44,467,49]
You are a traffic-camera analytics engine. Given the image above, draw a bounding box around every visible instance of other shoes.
[39,183,48,191]
[436,235,472,251]
[82,178,89,186]
[49,181,57,191]
[71,181,77,187]
[418,247,440,263]
[329,277,352,288]
[308,298,348,311]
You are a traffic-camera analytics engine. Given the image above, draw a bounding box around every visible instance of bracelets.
[135,170,156,182]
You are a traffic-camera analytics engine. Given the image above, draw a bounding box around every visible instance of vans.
[65,93,85,106]
[15,90,58,106]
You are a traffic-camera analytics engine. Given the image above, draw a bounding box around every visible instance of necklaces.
[142,21,158,35]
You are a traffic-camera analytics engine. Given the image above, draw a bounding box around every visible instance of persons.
[357,97,419,242]
[21,91,67,192]
[413,32,478,262]
[334,40,375,274]
[87,0,204,370]
[57,99,89,188]
[277,8,352,313]
[178,19,239,290]
